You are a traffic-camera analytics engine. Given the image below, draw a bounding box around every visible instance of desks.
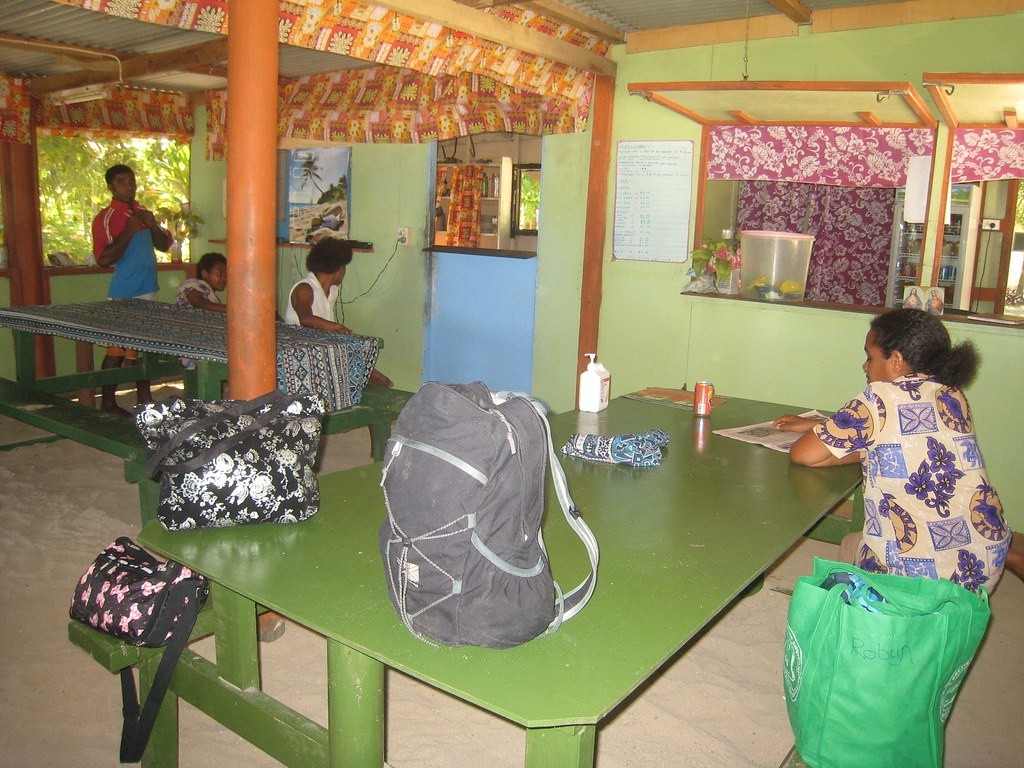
[0,298,400,464]
[134,388,864,768]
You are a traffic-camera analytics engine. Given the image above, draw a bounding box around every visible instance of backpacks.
[378,381,599,649]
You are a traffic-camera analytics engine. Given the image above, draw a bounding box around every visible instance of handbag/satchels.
[69,536,209,648]
[782,555,991,768]
[133,390,325,531]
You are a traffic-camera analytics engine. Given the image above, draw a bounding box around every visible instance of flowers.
[691,231,745,278]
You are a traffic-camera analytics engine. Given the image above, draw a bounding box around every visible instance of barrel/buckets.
[741,230,815,302]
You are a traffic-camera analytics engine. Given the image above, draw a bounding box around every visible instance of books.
[966,312,1024,326]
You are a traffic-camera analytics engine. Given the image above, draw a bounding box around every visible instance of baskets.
[716,269,740,295]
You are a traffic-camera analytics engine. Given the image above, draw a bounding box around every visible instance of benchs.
[776,745,809,768]
[353,385,417,464]
[67,576,273,768]
[1,377,161,529]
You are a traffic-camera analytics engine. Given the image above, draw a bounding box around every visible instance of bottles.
[481,172,488,197]
[928,290,943,314]
[717,228,739,295]
[903,288,922,309]
[490,215,498,233]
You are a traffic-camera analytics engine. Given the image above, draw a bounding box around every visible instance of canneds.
[939,267,956,280]
[694,381,714,416]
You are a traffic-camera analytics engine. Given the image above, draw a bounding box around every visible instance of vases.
[715,267,740,294]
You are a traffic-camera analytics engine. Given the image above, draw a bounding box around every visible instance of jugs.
[489,173,500,198]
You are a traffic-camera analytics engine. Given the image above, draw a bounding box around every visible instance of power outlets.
[982,219,1001,231]
[397,227,413,246]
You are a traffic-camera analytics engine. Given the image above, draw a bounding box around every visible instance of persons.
[175,252,227,371]
[91,165,174,419]
[284,237,394,390]
[903,288,923,310]
[925,290,944,315]
[773,308,1024,603]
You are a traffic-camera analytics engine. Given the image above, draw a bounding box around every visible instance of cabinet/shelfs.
[434,157,513,250]
[884,184,981,311]
